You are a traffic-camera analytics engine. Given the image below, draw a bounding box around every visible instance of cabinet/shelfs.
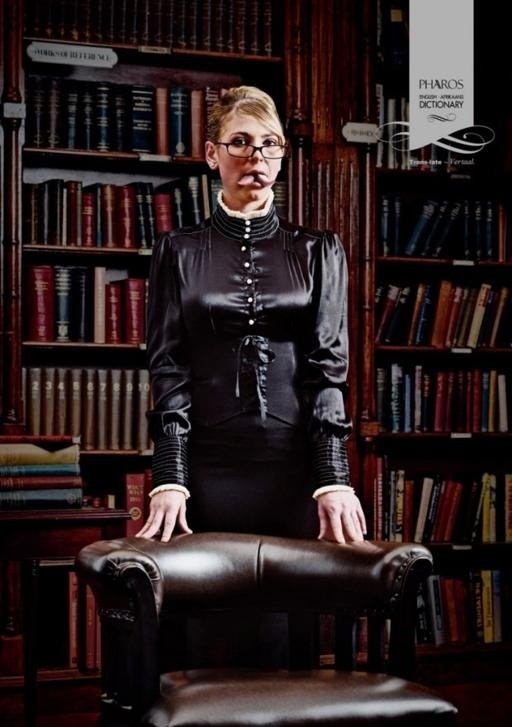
[0,1,512,688]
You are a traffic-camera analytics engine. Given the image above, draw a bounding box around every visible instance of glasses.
[214,143,288,159]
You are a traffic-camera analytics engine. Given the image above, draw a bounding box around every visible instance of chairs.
[72,530,462,726]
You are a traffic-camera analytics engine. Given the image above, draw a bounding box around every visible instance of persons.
[0,1,512,684]
